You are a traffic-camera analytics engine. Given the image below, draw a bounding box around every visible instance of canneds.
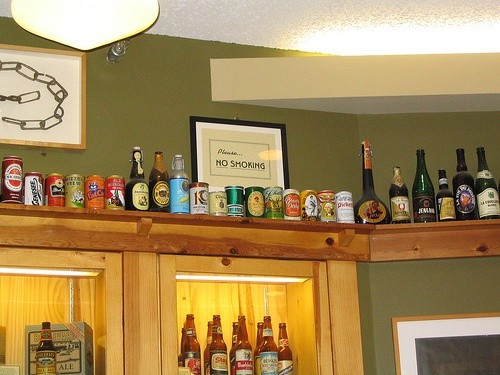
[189,182,356,224]
[1,156,125,210]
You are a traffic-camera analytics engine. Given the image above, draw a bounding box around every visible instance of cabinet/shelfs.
[1,247,365,375]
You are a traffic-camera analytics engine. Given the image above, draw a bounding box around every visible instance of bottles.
[451,147,479,221]
[410,149,437,222]
[169,153,192,214]
[388,165,411,224]
[472,146,500,220]
[350,141,391,225]
[125,146,151,211]
[147,150,171,213]
[178,312,294,375]
[435,167,457,222]
[35,322,56,375]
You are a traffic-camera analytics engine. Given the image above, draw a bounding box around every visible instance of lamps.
[10,0,160,52]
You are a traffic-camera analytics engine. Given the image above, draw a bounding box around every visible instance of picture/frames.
[190,115,290,191]
[392,311,500,375]
[0,43,87,150]
